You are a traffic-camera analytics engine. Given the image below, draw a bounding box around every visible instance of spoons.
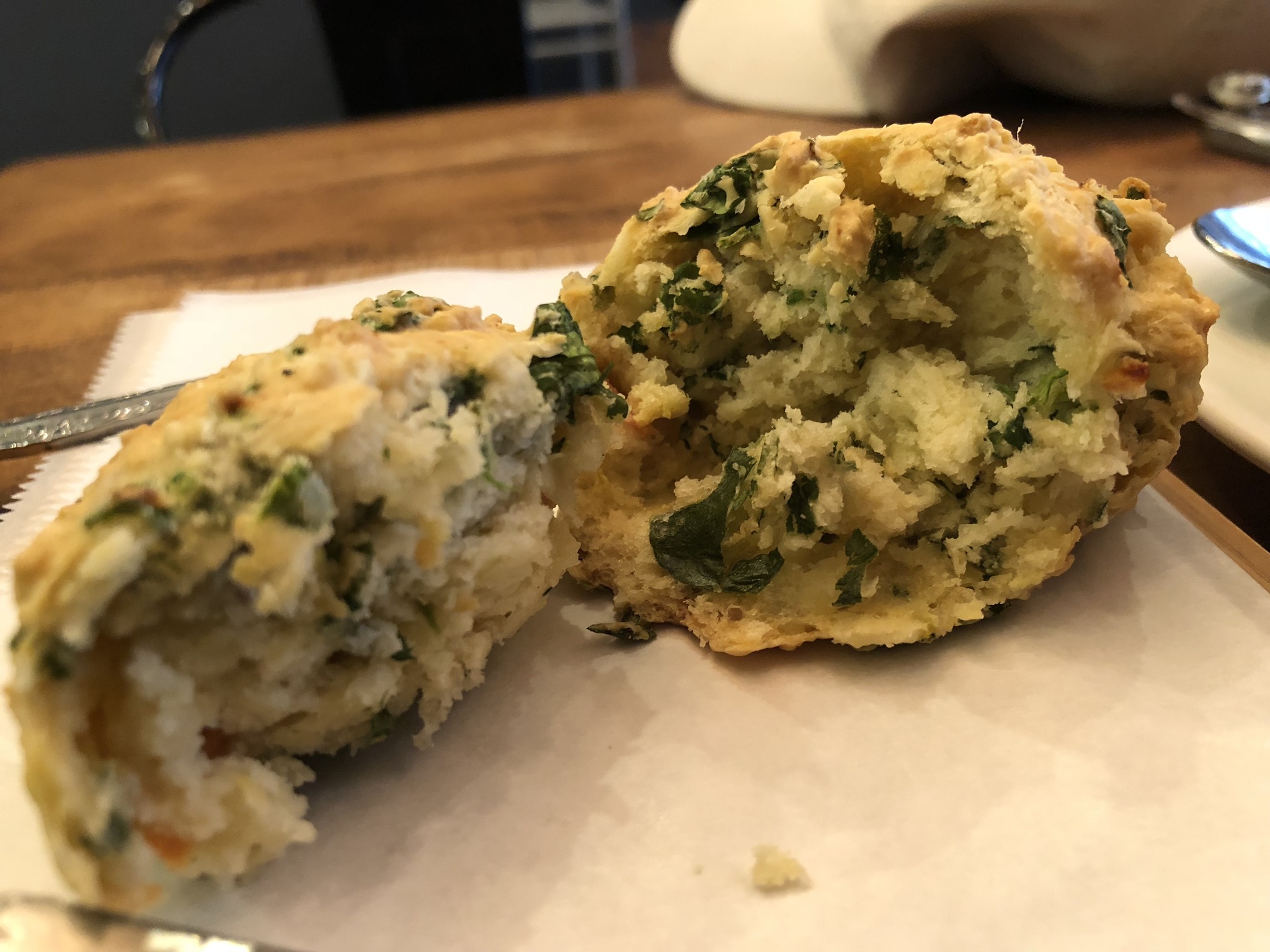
[1189,203,1270,290]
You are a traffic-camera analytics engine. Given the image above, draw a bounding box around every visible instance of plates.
[1156,190,1270,472]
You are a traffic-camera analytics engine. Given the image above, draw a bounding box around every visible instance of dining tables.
[0,84,1270,952]
